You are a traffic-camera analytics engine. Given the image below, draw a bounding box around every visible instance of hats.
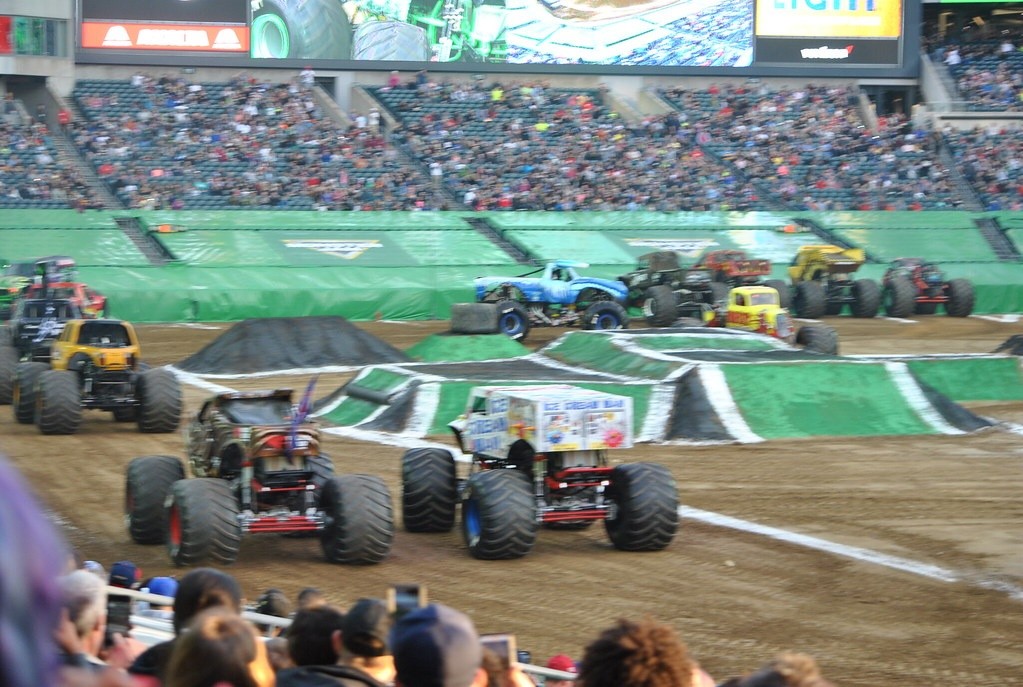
[147,577,179,597]
[110,561,135,586]
[546,654,578,673]
[390,602,481,687]
[340,599,393,657]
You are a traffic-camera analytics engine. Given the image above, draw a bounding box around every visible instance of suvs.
[785,243,883,323]
[883,256,978,321]
[682,250,778,314]
[1,255,116,401]
[126,385,398,566]
[472,255,637,339]
[398,383,679,562]
[614,249,717,329]
[698,285,841,360]
[9,314,185,430]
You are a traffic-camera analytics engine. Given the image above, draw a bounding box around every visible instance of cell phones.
[106,595,132,647]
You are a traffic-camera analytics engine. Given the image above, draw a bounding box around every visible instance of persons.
[504,0,755,64]
[1,36,1022,212]
[1,454,834,687]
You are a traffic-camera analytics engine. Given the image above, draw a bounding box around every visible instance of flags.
[288,381,315,451]
